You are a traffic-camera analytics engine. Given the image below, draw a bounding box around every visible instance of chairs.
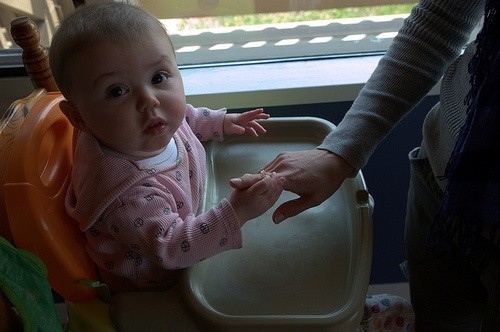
[0,17,375,332]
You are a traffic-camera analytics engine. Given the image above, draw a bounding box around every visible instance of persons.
[48,2,289,332]
[226,1,500,332]
